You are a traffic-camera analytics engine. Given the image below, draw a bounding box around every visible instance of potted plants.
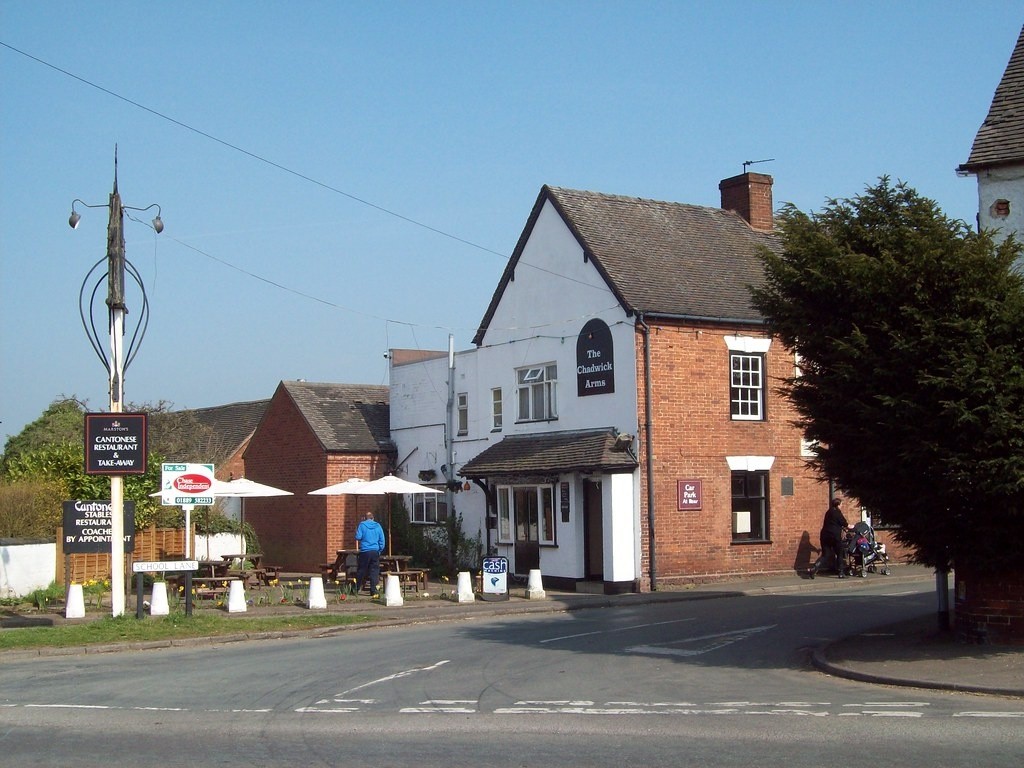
[444,479,463,495]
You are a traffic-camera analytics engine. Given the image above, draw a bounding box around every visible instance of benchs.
[381,571,423,593]
[408,567,431,590]
[227,569,266,590]
[192,577,239,600]
[251,566,282,587]
[165,574,184,601]
[320,563,346,584]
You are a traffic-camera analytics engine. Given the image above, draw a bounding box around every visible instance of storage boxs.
[732,512,751,533]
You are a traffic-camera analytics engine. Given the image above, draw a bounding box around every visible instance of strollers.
[842,521,892,577]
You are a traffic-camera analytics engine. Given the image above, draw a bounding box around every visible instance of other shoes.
[806,568,816,579]
[370,591,379,595]
[839,573,849,579]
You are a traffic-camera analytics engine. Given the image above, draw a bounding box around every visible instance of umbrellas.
[306,473,443,556]
[150,475,293,570]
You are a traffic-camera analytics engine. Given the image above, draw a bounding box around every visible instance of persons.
[355,512,386,596]
[807,498,855,580]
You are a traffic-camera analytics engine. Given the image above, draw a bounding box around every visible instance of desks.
[221,554,264,568]
[335,549,360,571]
[196,561,232,600]
[379,556,413,581]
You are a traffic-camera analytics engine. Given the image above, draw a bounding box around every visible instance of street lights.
[68,143,166,618]
